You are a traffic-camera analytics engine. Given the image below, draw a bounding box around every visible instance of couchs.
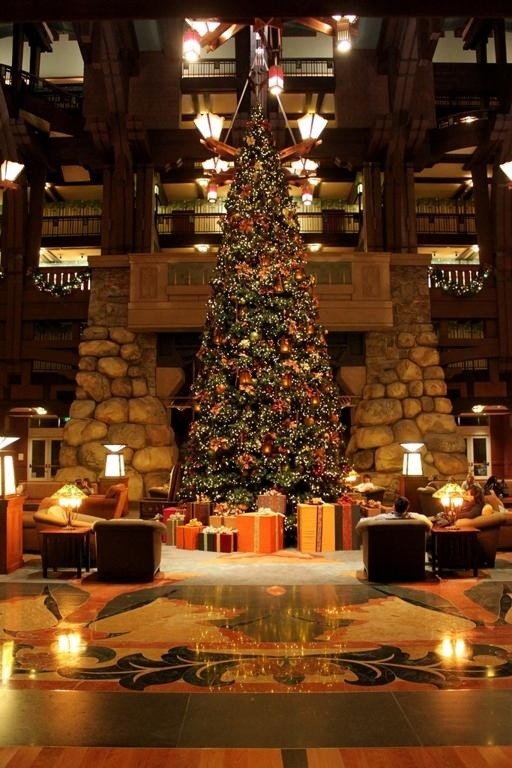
[17,480,71,508]
[416,478,512,515]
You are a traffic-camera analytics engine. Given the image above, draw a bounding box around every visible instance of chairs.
[355,519,432,582]
[94,518,167,583]
[24,481,128,552]
[455,510,512,568]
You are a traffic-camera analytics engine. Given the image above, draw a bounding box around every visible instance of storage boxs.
[160,490,362,555]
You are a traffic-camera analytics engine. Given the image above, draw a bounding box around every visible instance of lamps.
[398,440,425,477]
[100,442,128,478]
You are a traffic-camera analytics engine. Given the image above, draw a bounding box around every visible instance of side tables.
[39,525,92,579]
[431,525,480,580]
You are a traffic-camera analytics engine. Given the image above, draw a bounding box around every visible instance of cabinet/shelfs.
[97,476,129,516]
[0,496,25,574]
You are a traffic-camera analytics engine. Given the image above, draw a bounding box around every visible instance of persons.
[355,496,433,534]
[432,473,508,520]
[75,478,94,495]
[350,474,374,493]
